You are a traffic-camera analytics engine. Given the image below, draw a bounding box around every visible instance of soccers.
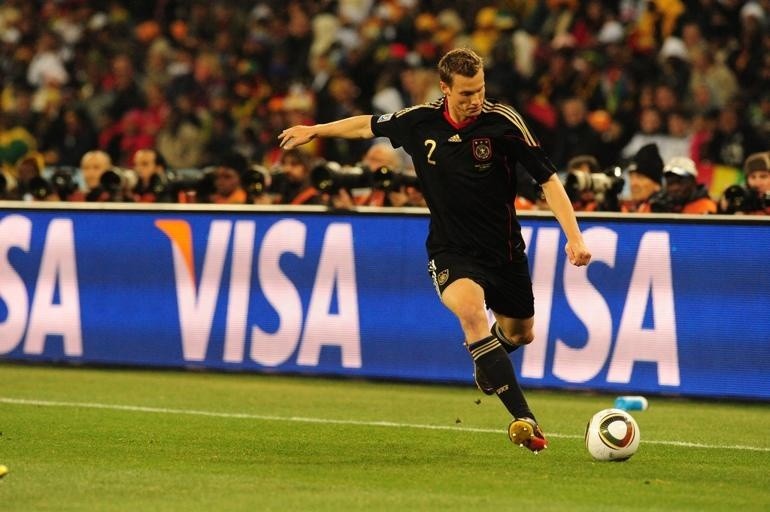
[585,408,640,461]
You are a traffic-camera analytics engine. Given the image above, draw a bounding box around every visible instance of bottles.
[614,395,649,410]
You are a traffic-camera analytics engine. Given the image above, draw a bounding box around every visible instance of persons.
[0,1,770,215]
[277,50,592,453]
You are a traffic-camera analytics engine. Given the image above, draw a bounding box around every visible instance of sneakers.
[464,340,497,397]
[506,416,547,453]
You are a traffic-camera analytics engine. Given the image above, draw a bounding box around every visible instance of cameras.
[651,193,676,212]
[725,184,759,211]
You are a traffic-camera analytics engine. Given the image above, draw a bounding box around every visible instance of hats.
[626,140,769,179]
